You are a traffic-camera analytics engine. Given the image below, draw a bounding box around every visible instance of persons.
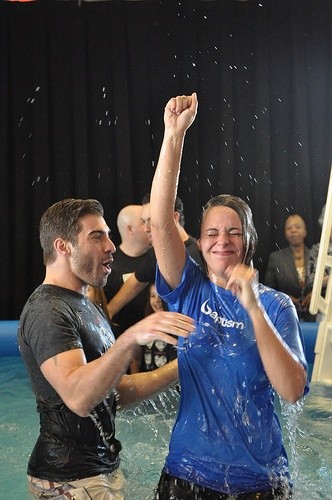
[129,280,180,376]
[87,204,152,377]
[105,190,208,394]
[16,197,197,500]
[262,210,316,322]
[300,203,332,321]
[148,91,308,500]
